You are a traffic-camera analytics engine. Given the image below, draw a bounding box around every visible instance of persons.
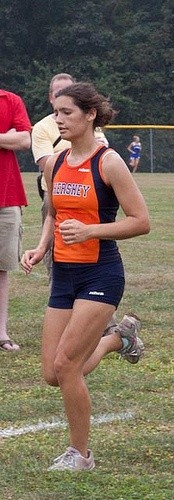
[31,73,119,337]
[21,81,151,470]
[128,135,142,174]
[0,89,32,351]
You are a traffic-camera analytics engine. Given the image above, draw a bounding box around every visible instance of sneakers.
[114,313,145,363]
[47,446,95,471]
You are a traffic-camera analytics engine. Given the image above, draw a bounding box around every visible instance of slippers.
[0,340,14,351]
